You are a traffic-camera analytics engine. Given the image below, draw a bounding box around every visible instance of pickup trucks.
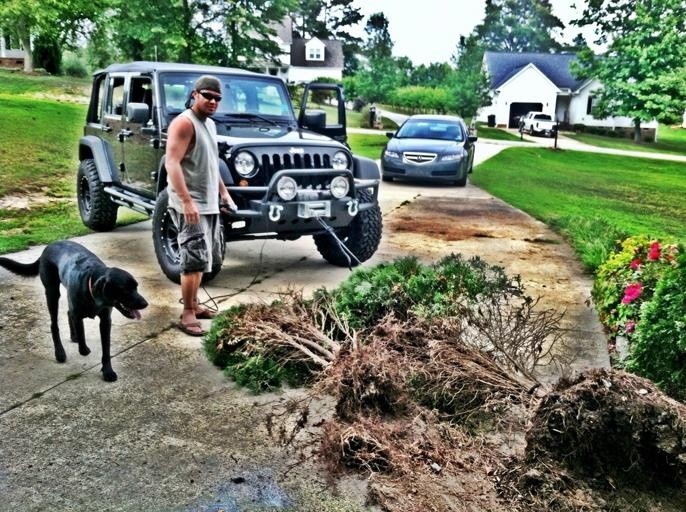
[517,111,560,139]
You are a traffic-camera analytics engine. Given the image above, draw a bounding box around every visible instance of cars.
[512,113,526,127]
[378,111,481,188]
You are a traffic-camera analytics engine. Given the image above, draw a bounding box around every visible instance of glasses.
[197,89,222,102]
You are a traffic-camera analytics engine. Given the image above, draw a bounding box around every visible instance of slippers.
[179,308,214,319]
[178,318,208,337]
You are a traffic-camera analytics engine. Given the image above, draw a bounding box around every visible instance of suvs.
[75,61,384,286]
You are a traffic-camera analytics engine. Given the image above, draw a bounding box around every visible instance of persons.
[164,76,237,336]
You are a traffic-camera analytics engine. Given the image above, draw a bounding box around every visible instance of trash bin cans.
[488,115,496,127]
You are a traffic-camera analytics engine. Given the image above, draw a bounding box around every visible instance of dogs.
[1,240,150,381]
